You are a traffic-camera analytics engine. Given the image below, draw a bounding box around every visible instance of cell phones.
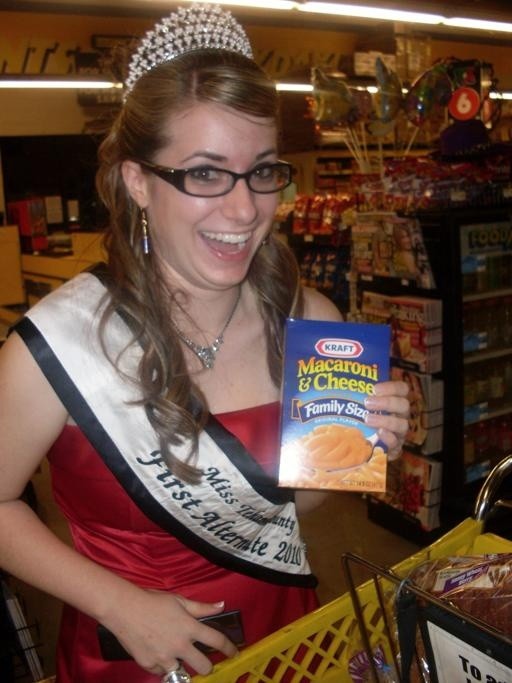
[96,608,247,662]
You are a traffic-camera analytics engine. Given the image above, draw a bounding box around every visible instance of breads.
[385,553,512,683]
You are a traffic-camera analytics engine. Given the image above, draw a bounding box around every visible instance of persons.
[1,0,410,683]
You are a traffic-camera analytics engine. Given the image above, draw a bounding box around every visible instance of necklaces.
[170,284,242,370]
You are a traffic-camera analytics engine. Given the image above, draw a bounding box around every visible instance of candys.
[306,56,452,176]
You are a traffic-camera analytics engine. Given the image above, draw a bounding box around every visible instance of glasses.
[117,158,292,198]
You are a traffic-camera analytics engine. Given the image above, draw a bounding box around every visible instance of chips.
[279,422,387,492]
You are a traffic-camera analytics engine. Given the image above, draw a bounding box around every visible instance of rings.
[165,664,192,683]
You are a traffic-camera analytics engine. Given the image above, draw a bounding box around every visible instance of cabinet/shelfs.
[348,196,512,546]
